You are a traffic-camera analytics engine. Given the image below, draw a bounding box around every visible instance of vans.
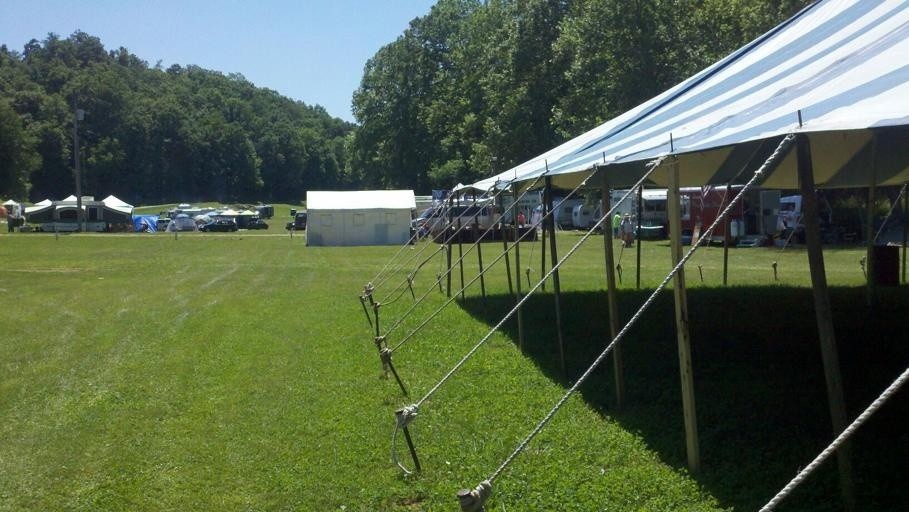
[780,195,809,237]
[413,204,490,233]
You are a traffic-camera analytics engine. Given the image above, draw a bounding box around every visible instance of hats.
[623,213,631,217]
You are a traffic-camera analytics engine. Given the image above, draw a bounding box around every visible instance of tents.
[129,203,256,232]
[305,189,417,245]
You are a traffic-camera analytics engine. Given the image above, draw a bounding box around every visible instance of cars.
[201,219,238,234]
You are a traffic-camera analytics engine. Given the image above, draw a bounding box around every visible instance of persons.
[518,208,549,235]
[613,211,636,247]
[781,217,787,228]
[7,211,15,232]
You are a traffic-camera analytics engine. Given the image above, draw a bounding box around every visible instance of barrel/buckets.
[865,242,902,299]
[291,209,296,217]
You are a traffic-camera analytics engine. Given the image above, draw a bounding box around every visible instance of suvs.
[247,218,269,230]
